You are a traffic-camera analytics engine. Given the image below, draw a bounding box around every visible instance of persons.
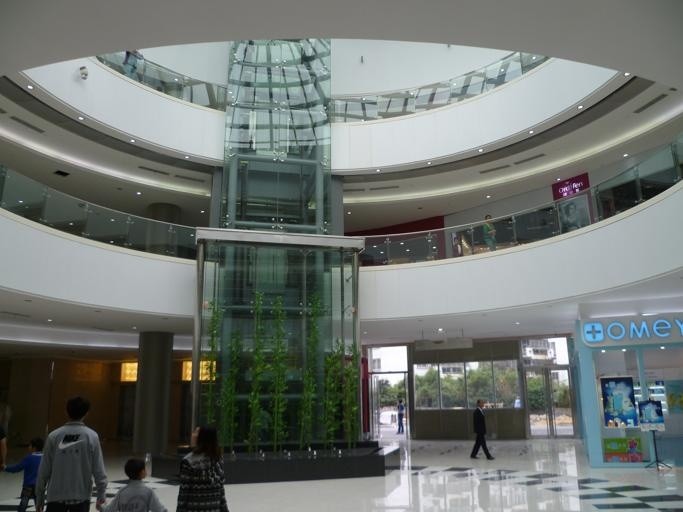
[394,398,405,435]
[171,422,231,512]
[0,424,9,474]
[94,457,169,511]
[559,203,582,232]
[32,395,109,511]
[480,215,498,252]
[452,236,464,257]
[1,437,43,512]
[468,398,495,459]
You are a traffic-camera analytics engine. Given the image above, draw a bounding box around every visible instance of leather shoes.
[488,457,494,459]
[471,455,478,458]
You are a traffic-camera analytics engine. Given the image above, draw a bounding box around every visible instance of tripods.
[645,431,672,471]
